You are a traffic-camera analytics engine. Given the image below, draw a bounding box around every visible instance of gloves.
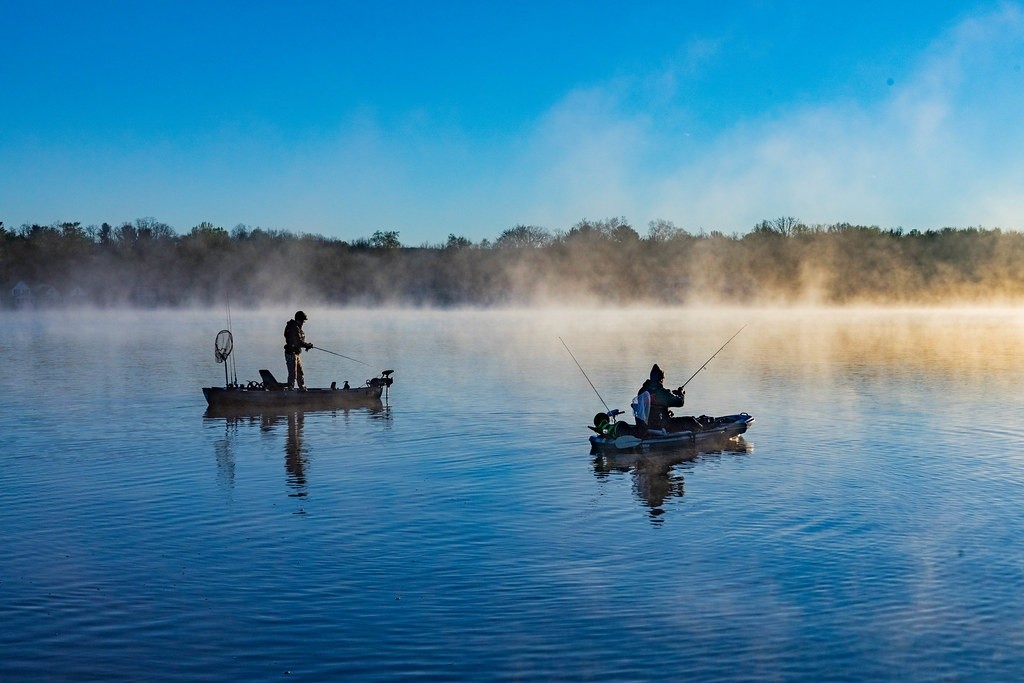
[678,387,685,395]
[304,343,313,351]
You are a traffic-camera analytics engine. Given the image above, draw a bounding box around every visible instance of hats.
[296,311,309,320]
[650,363,664,380]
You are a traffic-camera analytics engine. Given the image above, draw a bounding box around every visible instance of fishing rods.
[306,343,379,371]
[662,316,752,408]
[556,333,622,428]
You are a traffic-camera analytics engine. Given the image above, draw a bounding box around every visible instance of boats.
[201,367,397,410]
[587,404,755,455]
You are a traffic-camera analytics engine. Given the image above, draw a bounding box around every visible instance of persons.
[633,365,685,436]
[284,312,312,391]
